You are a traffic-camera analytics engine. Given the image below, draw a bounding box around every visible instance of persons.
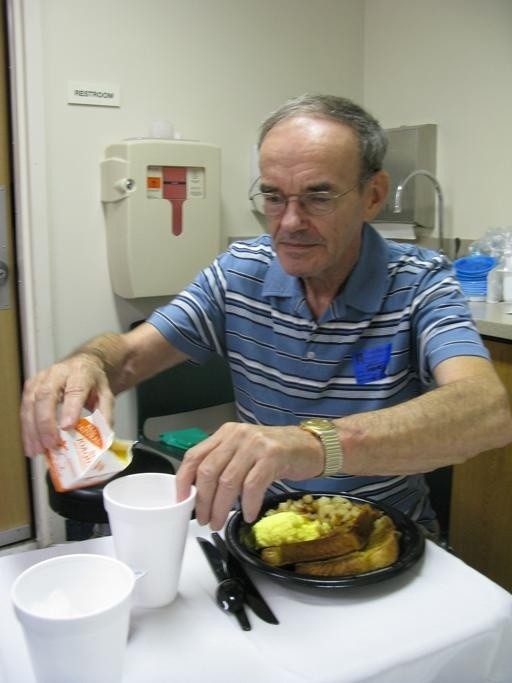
[18,89,512,545]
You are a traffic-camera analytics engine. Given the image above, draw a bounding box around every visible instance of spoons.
[216,552,245,612]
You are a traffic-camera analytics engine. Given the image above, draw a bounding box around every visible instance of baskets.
[454,255,496,297]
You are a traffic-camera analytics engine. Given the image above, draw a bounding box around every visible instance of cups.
[102,472,196,608]
[14,554,136,683]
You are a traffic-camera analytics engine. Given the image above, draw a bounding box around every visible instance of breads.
[250,494,377,566]
[296,516,399,577]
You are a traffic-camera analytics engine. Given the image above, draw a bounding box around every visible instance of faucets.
[393,170,446,256]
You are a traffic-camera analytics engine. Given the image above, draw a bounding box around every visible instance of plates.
[224,490,425,590]
[452,255,496,297]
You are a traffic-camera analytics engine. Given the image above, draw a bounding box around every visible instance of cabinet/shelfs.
[447,300,512,594]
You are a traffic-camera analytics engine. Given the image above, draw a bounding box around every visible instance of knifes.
[196,537,251,631]
[213,531,279,625]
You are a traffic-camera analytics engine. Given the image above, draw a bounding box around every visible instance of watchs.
[297,417,344,478]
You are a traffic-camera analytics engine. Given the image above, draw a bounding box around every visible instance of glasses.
[249,176,363,217]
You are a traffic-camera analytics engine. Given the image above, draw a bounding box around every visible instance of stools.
[46,449,175,540]
